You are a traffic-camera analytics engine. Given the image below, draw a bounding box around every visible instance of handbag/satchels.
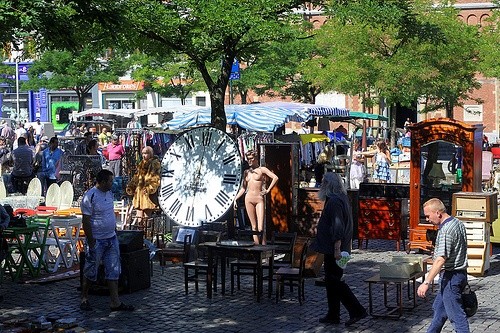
[461,283,478,317]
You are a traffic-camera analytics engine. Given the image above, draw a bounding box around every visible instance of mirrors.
[407,119,486,225]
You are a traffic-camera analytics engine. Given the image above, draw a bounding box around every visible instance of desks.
[364,271,434,315]
[1,196,45,211]
[199,241,280,304]
[35,217,82,273]
[2,224,40,282]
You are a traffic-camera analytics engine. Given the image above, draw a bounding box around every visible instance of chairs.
[228,229,263,296]
[262,232,298,297]
[157,228,198,266]
[0,177,133,284]
[275,239,308,306]
[183,229,221,299]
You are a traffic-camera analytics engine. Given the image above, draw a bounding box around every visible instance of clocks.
[157,126,242,227]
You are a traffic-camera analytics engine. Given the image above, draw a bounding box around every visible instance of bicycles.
[448,146,461,173]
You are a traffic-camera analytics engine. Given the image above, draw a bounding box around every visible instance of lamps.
[428,162,446,188]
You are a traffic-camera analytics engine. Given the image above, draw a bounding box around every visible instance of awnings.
[330,111,388,122]
[163,102,350,132]
[145,105,196,114]
[75,108,144,118]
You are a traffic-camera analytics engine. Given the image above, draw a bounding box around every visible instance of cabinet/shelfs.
[359,196,406,252]
[257,141,300,240]
[298,187,355,251]
[452,192,498,277]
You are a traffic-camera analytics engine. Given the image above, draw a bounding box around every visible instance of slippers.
[111,302,141,313]
[81,300,90,310]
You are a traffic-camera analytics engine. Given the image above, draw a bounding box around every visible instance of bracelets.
[334,248,340,250]
[424,280,429,285]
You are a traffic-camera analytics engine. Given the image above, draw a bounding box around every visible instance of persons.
[310,172,368,326]
[65,122,125,176]
[0,119,64,193]
[126,146,160,222]
[234,149,278,246]
[417,198,470,333]
[80,169,136,312]
[297,116,414,189]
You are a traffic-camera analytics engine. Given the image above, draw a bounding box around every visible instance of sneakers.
[319,315,340,324]
[345,310,368,326]
